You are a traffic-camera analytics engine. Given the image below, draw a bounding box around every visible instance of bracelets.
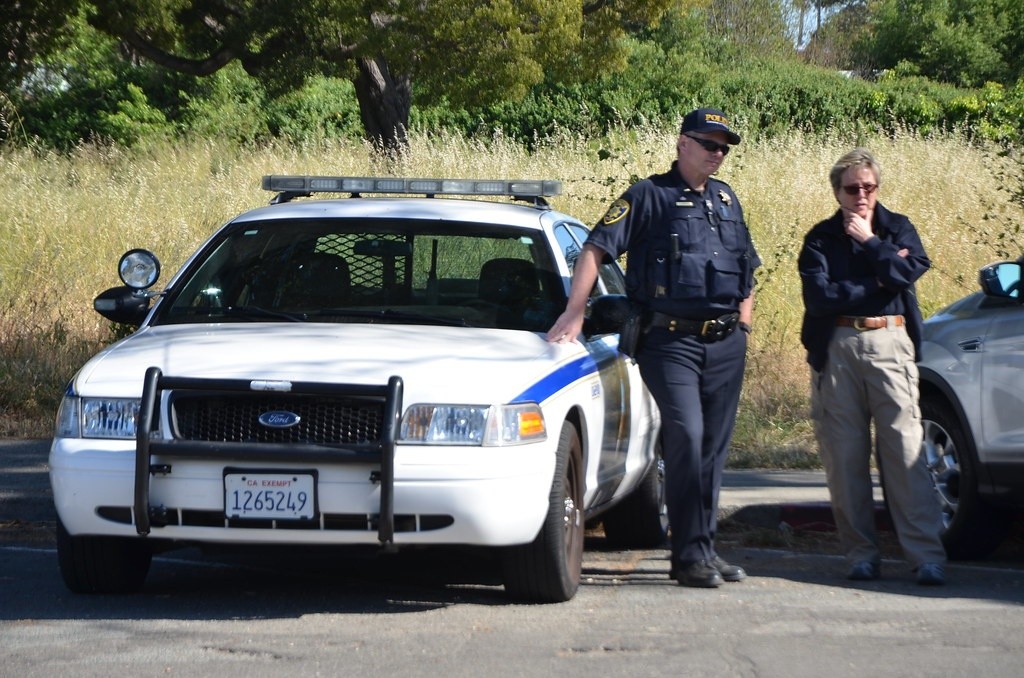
[738,321,752,334]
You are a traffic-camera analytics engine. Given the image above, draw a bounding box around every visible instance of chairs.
[460,258,540,308]
[264,253,351,311]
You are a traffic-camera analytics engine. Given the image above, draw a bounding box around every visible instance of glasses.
[680,131,730,157]
[840,183,880,196]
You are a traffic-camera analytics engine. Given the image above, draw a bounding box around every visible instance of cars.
[47,174,670,604]
[876,252,1023,553]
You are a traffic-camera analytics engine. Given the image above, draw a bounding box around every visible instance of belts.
[645,309,745,344]
[828,314,908,333]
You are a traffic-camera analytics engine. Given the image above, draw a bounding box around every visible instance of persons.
[545,107,767,588]
[796,147,947,587]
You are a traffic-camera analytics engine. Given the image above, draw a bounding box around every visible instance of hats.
[679,108,742,147]
